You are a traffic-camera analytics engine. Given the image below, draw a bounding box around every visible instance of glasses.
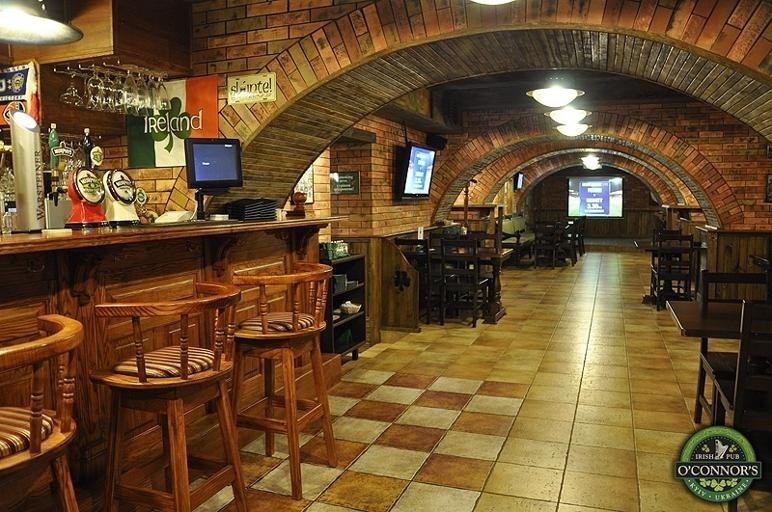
[0,0,84,48]
[523,67,603,171]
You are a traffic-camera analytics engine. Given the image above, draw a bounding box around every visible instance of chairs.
[0,311,89,512]
[230,257,339,501]
[384,213,586,328]
[91,277,251,512]
[629,222,772,512]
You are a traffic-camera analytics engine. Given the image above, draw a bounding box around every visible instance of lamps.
[384,213,586,328]
[230,257,339,501]
[523,67,603,171]
[0,311,89,512]
[91,277,251,512]
[0,0,84,48]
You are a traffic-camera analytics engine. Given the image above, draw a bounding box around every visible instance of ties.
[514,171,524,190]
[183,138,243,189]
[400,144,436,200]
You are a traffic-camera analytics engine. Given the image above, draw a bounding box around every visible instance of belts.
[54,63,174,120]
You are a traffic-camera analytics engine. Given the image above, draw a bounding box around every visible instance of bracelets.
[340,303,362,315]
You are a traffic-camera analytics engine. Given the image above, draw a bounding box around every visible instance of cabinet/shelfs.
[47,123,104,174]
[8,0,194,81]
[316,250,368,364]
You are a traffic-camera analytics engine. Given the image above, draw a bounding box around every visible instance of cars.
[326,240,349,256]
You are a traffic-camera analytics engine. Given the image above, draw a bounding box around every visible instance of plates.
[8,0,194,81]
[316,250,368,364]
